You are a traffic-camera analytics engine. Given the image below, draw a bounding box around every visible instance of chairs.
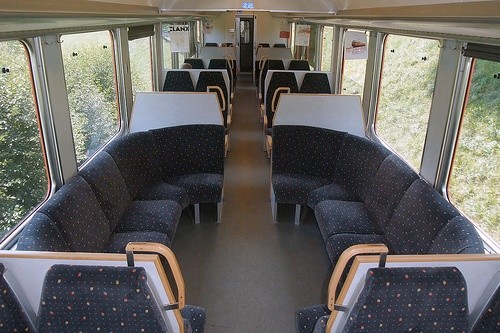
[255,43,271,58]
[288,60,311,71]
[264,72,299,159]
[0,259,173,333]
[342,265,471,333]
[257,60,285,123]
[206,43,217,47]
[196,71,230,158]
[208,59,234,104]
[184,59,205,69]
[273,43,286,48]
[220,43,233,47]
[163,71,195,92]
[299,73,331,94]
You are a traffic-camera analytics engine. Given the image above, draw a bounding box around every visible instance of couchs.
[269,124,500,333]
[16,122,227,333]
